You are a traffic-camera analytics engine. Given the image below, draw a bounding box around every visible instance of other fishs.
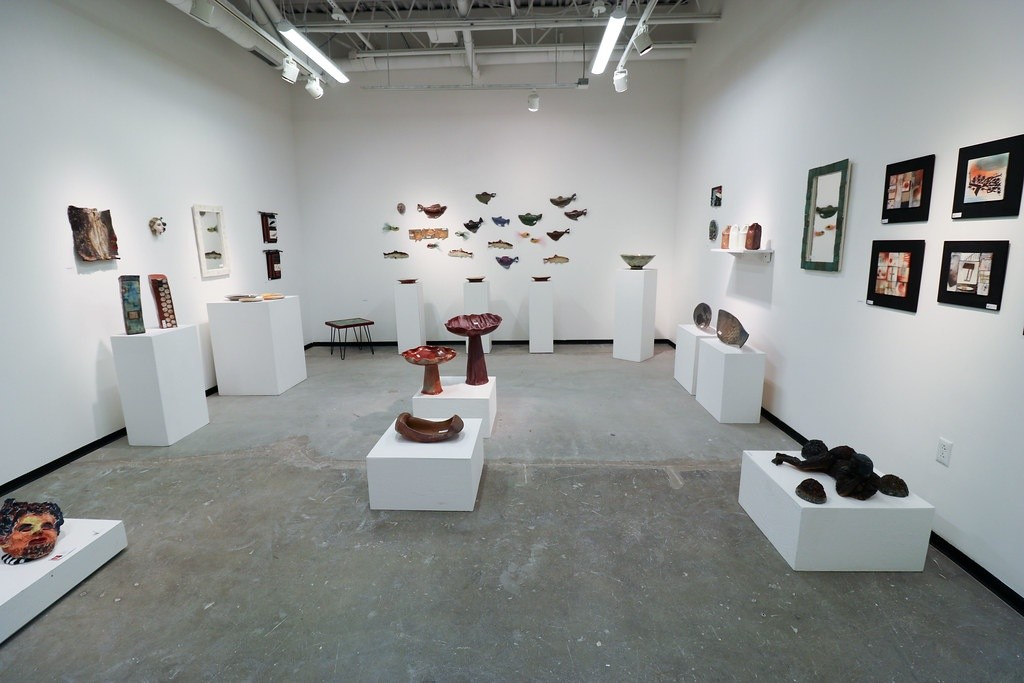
[379,191,586,265]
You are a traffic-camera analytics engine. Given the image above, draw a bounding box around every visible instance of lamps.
[632,20,653,56]
[613,65,627,93]
[590,7,627,75]
[528,88,541,112]
[277,19,350,100]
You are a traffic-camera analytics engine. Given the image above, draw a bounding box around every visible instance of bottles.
[720,222,771,251]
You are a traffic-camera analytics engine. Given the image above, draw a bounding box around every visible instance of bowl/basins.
[621,254,656,269]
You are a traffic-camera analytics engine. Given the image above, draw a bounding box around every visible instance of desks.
[325,318,375,360]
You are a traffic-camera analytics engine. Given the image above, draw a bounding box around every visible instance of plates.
[397,279,417,284]
[693,302,712,330]
[225,295,256,300]
[466,277,485,282]
[531,276,551,281]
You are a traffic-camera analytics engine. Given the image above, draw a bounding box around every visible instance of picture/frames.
[881,153,936,223]
[865,239,925,313]
[951,134,1024,220]
[937,240,1010,312]
[192,206,230,278]
[800,159,852,273]
